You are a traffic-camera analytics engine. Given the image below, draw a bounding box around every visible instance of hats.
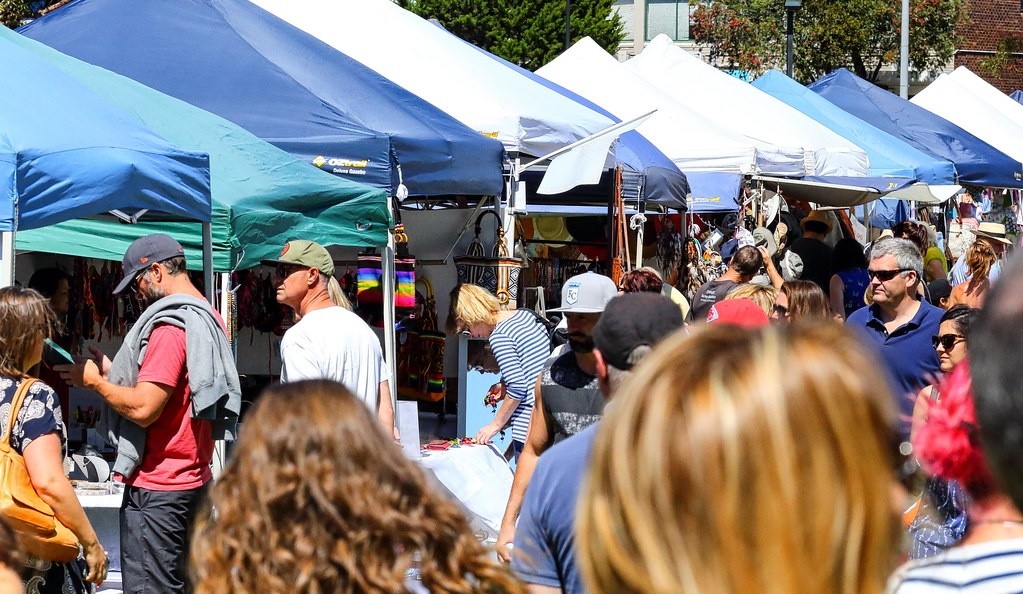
[970,221,1013,245]
[591,290,685,371]
[800,209,833,235]
[705,297,768,332]
[926,279,952,307]
[752,227,778,259]
[544,271,618,313]
[260,239,335,278]
[530,215,573,249]
[112,233,185,294]
[779,251,804,283]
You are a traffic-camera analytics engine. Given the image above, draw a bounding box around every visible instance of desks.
[69,439,521,594]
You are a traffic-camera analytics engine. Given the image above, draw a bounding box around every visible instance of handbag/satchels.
[357,195,415,308]
[948,196,975,259]
[454,209,523,312]
[0,378,81,564]
[517,307,569,354]
[396,278,447,403]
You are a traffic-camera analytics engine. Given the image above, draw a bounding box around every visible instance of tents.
[0,1,1023,440]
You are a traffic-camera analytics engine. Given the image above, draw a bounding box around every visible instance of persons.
[53,233,241,594]
[260,238,397,442]
[684,245,785,322]
[0,287,111,594]
[497,198,1023,594]
[842,237,951,428]
[188,379,526,594]
[949,221,1011,287]
[496,271,617,563]
[447,281,553,465]
[28,265,73,414]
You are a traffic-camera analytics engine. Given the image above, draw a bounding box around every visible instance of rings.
[105,560,111,567]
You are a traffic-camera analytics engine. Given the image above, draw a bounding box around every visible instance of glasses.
[868,268,905,282]
[932,333,966,350]
[130,266,151,294]
[461,324,471,337]
[279,265,304,278]
[773,303,790,318]
